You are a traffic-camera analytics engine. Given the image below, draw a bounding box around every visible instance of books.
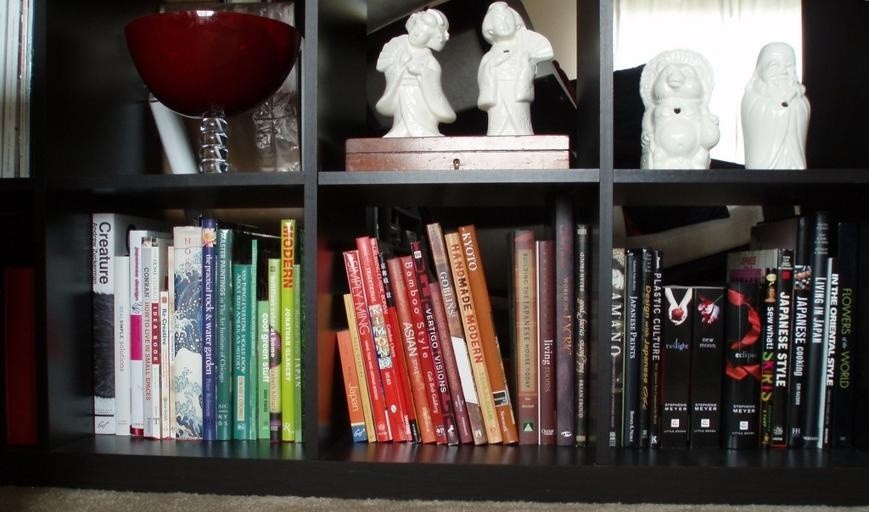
[91,213,306,444]
[335,199,592,449]
[0,0,33,178]
[607,210,856,450]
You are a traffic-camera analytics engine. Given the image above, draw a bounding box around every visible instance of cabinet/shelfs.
[0,0,869,504]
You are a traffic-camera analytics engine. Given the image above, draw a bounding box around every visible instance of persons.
[477,0,553,137]
[741,41,812,170]
[374,8,457,138]
